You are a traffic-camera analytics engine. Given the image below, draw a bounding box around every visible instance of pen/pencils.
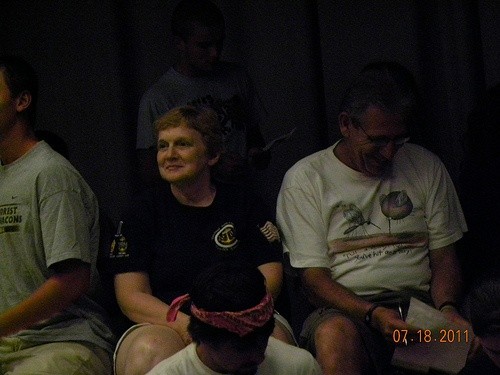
[396,296,408,346]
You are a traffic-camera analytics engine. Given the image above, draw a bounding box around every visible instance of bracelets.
[364,305,377,326]
[438,300,455,312]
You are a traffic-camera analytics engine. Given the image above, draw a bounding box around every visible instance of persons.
[143,261,325,375]
[276,86,500,375]
[106,105,298,375]
[0,57,119,375]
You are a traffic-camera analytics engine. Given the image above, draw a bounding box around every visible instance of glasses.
[359,123,411,147]
[210,134,247,153]
